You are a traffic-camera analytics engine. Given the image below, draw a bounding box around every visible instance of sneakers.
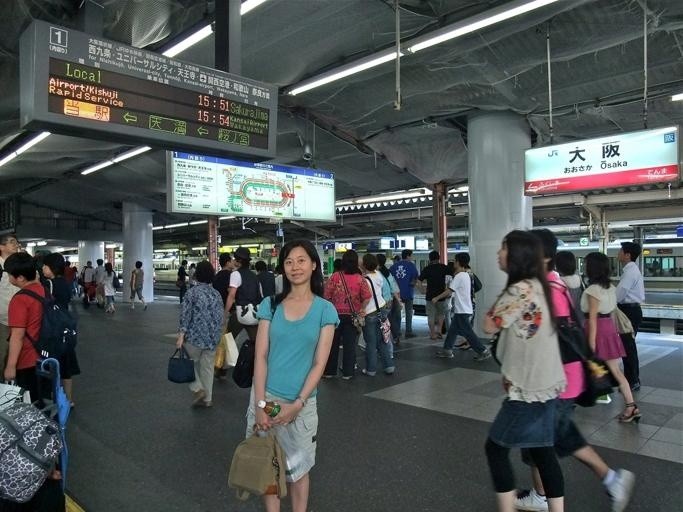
[341,374,353,380]
[472,351,492,361]
[340,363,358,370]
[188,389,206,406]
[129,305,135,310]
[605,465,637,512]
[514,485,552,512]
[405,333,416,339]
[321,374,333,379]
[431,349,454,359]
[143,303,148,311]
[393,336,401,345]
[384,367,396,374]
[390,355,394,359]
[198,400,212,408]
[109,309,116,314]
[362,368,376,376]
[105,307,111,312]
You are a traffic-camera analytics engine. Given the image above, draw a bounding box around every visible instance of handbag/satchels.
[233,294,281,388]
[544,281,593,364]
[167,345,196,383]
[615,307,633,334]
[214,337,226,368]
[224,326,239,369]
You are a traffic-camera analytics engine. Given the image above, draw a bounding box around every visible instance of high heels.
[463,345,473,351]
[454,341,467,347]
[616,401,641,424]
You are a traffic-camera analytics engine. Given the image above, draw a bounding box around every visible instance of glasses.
[8,238,19,246]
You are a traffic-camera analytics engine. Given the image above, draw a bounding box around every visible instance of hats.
[232,245,253,264]
[454,250,473,270]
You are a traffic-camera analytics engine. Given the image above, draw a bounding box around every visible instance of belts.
[617,303,639,308]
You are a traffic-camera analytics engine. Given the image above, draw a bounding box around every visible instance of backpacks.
[233,338,255,390]
[570,321,617,406]
[233,271,264,326]
[14,285,79,370]
[474,276,482,292]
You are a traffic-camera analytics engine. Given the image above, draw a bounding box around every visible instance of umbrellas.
[40,359,70,495]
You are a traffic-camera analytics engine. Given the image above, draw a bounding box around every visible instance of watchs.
[297,396,307,408]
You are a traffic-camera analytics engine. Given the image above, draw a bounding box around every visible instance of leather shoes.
[618,382,639,394]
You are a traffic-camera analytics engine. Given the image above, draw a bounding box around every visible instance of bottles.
[258,401,295,424]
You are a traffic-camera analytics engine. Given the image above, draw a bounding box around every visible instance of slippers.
[437,332,444,339]
[430,335,437,340]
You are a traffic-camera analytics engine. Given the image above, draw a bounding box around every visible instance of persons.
[326,250,372,380]
[43,253,81,406]
[4,251,53,401]
[73,267,81,294]
[274,265,284,296]
[176,260,224,409]
[454,251,477,350]
[178,259,190,303]
[361,253,396,376]
[375,254,403,319]
[416,251,455,340]
[187,263,198,289]
[483,229,565,512]
[391,250,420,338]
[80,261,95,305]
[0,233,23,380]
[94,259,107,308]
[385,256,401,270]
[248,239,341,512]
[514,228,634,511]
[614,241,646,393]
[646,257,683,277]
[578,252,643,426]
[212,252,232,306]
[213,247,263,382]
[254,259,275,298]
[432,254,492,361]
[553,251,583,315]
[101,263,117,314]
[130,261,149,312]
[64,261,76,313]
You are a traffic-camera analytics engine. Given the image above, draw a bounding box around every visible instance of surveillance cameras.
[303,144,313,160]
